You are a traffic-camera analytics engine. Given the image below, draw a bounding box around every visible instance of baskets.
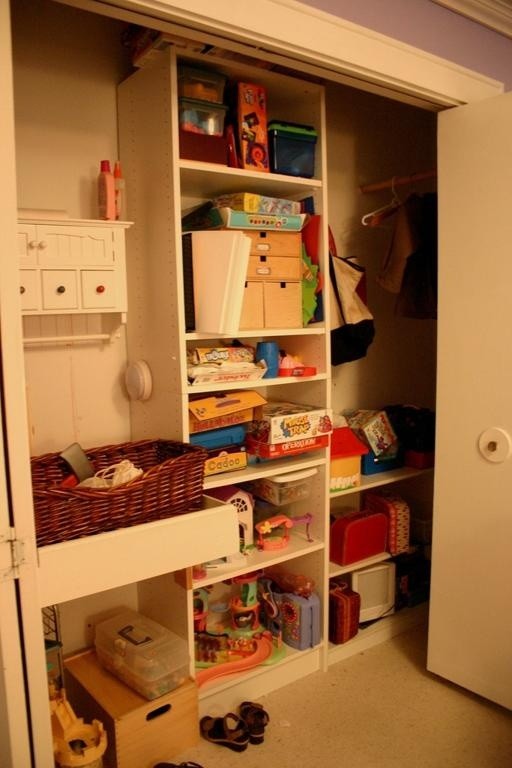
[30,436,205,545]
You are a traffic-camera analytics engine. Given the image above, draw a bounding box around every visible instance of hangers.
[361,175,400,227]
[370,175,422,229]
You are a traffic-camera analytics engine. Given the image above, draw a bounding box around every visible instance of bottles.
[96,158,128,221]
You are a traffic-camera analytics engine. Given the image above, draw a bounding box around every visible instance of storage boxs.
[179,98,229,138]
[264,118,318,178]
[178,63,229,104]
[58,652,203,768]
[91,607,191,692]
[224,223,306,331]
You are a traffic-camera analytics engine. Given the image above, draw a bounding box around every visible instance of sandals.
[240,701,271,746]
[198,713,248,753]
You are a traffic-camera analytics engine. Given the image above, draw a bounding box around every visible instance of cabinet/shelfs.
[117,54,327,710]
[327,465,431,665]
[17,217,136,312]
